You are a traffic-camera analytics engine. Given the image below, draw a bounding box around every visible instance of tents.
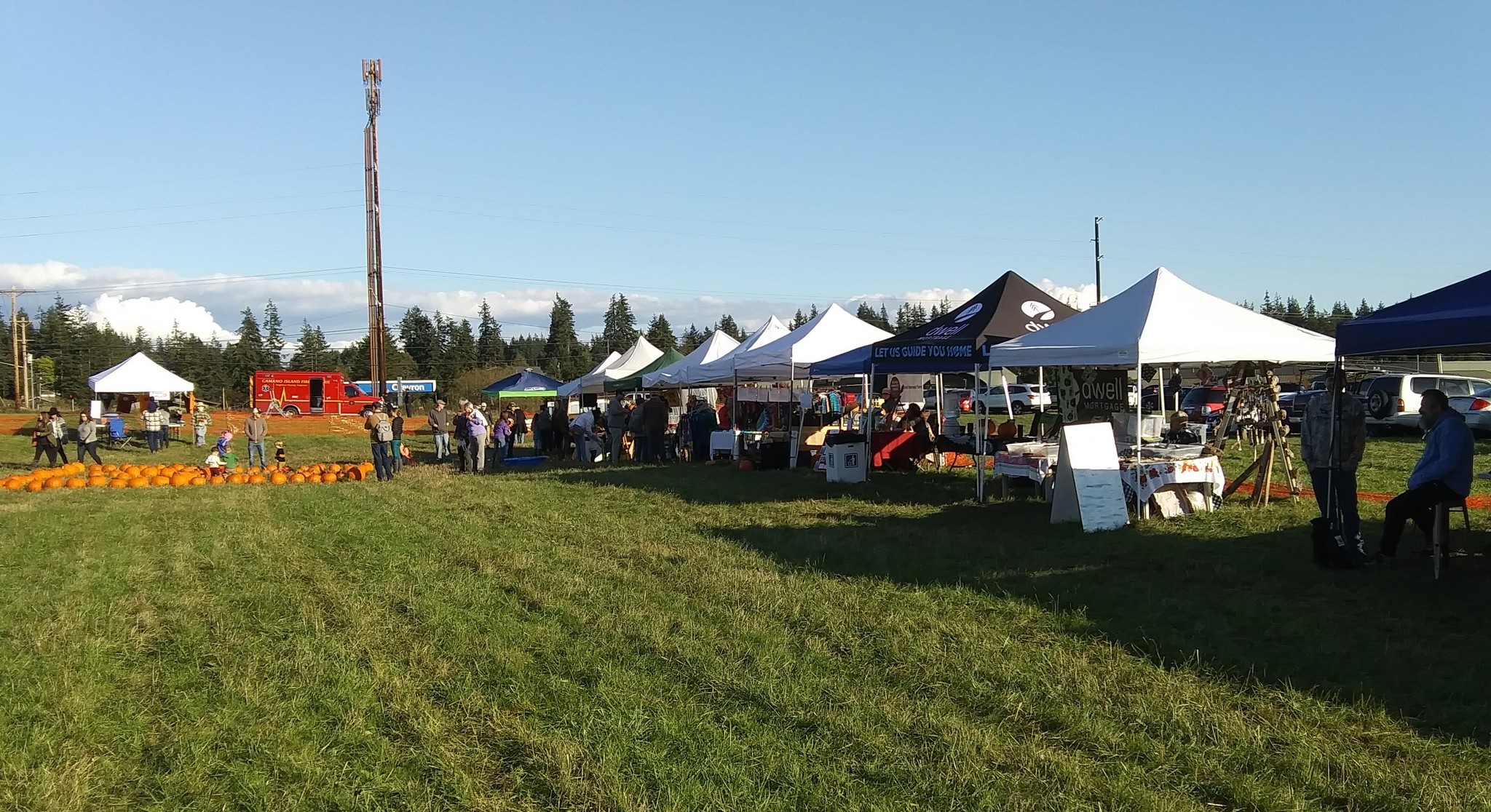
[88,352,195,446]
[480,268,1491,518]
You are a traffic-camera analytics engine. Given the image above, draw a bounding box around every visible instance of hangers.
[832,387,841,394]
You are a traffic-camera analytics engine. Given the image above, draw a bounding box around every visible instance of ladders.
[1213,360,1302,506]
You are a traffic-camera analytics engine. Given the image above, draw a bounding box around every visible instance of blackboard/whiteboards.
[1049,421,1130,533]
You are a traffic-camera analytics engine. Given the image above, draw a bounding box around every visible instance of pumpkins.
[737,457,752,471]
[979,419,1017,436]
[0,460,374,491]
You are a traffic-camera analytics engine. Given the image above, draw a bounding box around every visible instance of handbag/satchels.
[570,424,582,432]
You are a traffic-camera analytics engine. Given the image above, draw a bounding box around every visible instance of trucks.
[249,369,384,418]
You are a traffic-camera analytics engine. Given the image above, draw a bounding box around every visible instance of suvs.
[924,382,1059,415]
[1276,373,1491,438]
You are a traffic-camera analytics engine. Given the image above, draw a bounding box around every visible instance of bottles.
[847,418,852,430]
[967,423,973,435]
[943,393,961,421]
[902,421,907,432]
[1018,425,1023,438]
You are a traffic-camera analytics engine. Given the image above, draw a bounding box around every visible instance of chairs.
[915,436,940,474]
[109,418,137,448]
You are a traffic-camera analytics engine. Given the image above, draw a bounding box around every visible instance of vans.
[1178,385,1242,424]
[811,374,926,414]
[623,392,651,403]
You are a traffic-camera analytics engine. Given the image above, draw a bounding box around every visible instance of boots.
[398,458,403,471]
[392,459,399,475]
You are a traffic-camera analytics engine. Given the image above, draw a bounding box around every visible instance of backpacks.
[374,412,393,441]
[629,407,644,433]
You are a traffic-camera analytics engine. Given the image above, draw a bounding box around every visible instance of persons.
[274,440,286,471]
[364,388,944,481]
[77,410,103,466]
[26,407,69,470]
[1300,368,1375,563]
[191,401,212,447]
[141,402,170,455]
[205,431,240,481]
[244,408,268,469]
[1370,389,1475,567]
[1162,411,1198,445]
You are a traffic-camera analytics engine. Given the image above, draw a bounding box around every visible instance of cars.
[1205,390,1298,434]
[1127,384,1193,410]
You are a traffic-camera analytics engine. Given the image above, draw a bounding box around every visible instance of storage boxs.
[1161,423,1208,444]
[1129,443,1205,459]
[1006,442,1060,457]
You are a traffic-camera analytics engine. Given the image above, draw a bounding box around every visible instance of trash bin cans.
[825,433,867,483]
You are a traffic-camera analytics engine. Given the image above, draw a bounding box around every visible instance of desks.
[1120,453,1218,521]
[96,424,110,441]
[168,423,186,439]
[992,451,1058,504]
[710,430,735,461]
[830,430,927,472]
[936,434,1036,473]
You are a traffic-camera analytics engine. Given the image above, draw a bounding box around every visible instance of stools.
[1410,496,1471,580]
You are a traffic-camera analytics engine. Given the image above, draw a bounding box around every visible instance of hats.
[48,407,60,415]
[650,390,661,396]
[616,389,626,395]
[508,401,517,407]
[405,388,410,390]
[252,408,264,414]
[437,399,446,405]
[481,403,487,408]
[195,402,209,409]
[1325,367,1346,380]
[882,388,892,395]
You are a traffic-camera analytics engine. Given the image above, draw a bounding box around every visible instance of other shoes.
[1421,540,1450,555]
[459,468,472,475]
[1362,552,1397,569]
[1348,534,1368,562]
[445,457,452,462]
[472,469,485,476]
[436,458,442,465]
